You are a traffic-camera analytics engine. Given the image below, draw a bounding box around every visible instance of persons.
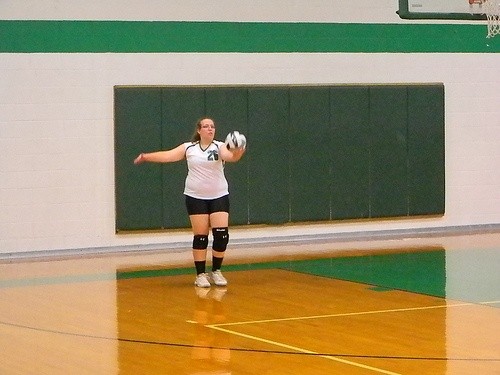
[193,283,232,375]
[133,117,245,289]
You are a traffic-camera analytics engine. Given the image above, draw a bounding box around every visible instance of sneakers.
[211,270,227,286]
[194,273,210,287]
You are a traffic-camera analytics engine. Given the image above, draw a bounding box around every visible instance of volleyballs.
[224,130,247,152]
